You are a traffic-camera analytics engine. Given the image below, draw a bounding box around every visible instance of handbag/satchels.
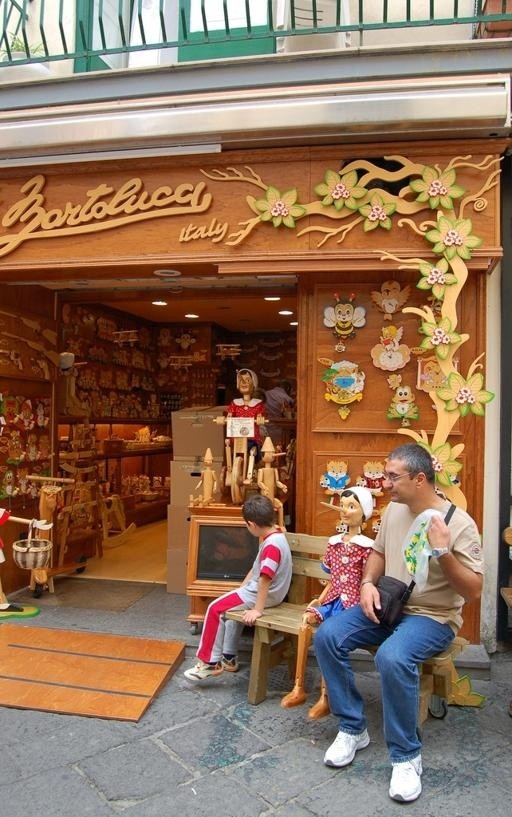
[373,575,410,632]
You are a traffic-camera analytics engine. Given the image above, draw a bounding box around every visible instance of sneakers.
[324,727,371,768]
[184,661,223,682]
[388,752,424,803]
[221,655,240,672]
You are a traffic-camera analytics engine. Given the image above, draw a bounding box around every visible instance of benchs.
[221,530,471,728]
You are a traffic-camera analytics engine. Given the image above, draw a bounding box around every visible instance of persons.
[183,493,293,686]
[226,366,267,486]
[258,378,295,444]
[256,434,290,532]
[187,446,219,509]
[0,506,55,614]
[280,481,383,721]
[311,440,487,807]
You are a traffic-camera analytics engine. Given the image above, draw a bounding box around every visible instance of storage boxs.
[165,404,229,596]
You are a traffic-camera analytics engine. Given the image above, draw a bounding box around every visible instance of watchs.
[430,545,449,561]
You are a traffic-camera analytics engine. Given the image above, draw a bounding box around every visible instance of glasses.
[382,470,413,482]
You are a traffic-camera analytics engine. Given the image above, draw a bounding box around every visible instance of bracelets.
[355,576,372,591]
